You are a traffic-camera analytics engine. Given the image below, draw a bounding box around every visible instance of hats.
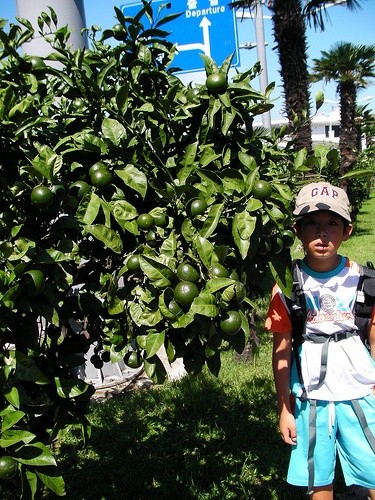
[290,181,351,224]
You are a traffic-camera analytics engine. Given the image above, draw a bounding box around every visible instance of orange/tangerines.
[10,23,295,368]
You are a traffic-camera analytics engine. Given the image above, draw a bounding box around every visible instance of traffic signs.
[117,0,241,78]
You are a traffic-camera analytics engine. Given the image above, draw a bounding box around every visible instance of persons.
[260,179,375,500]
